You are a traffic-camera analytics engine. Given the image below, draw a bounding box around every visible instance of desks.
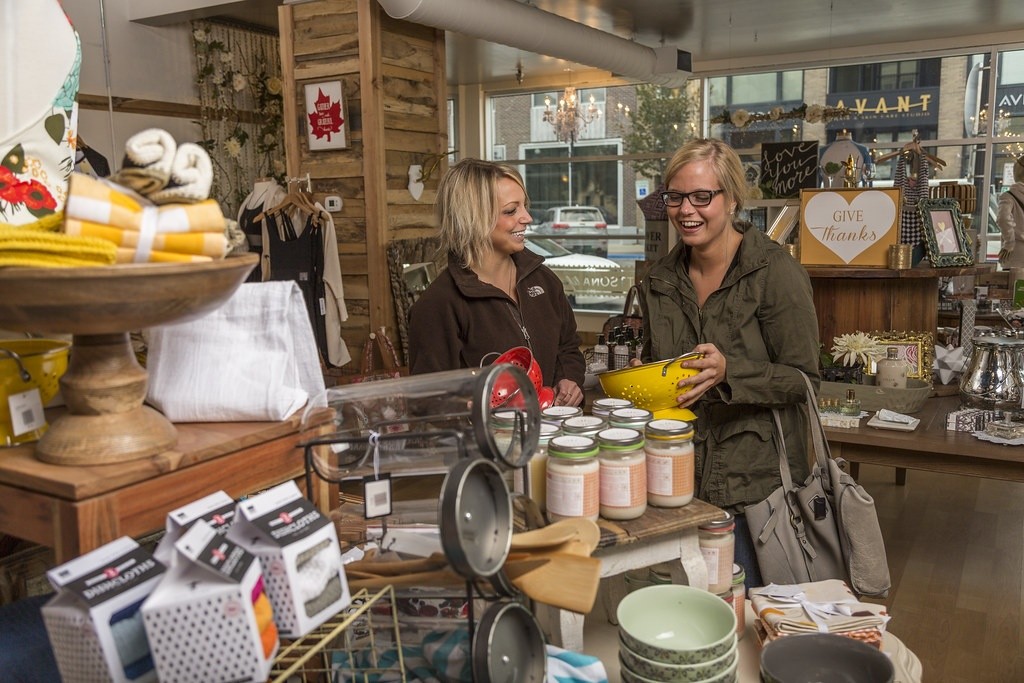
[551,498,726,655]
[823,384,1024,486]
[1,405,339,602]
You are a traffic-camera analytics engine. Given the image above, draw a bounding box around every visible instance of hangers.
[256,181,329,228]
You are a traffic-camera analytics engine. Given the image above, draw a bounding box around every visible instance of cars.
[523,229,624,272]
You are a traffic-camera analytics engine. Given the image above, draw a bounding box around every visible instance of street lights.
[543,91,591,207]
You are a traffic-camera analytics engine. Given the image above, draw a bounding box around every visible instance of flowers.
[832,332,878,366]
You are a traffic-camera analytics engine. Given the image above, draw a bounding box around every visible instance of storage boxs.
[43,486,351,682]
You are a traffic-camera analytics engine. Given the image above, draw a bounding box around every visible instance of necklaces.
[509,263,512,296]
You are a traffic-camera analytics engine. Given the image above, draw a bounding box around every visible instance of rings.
[568,392,572,396]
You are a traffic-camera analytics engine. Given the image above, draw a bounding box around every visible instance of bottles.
[876,348,908,389]
[936,326,1024,348]
[818,389,861,416]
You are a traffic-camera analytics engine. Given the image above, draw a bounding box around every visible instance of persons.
[407,158,586,430]
[998,154,1024,271]
[820,132,872,187]
[625,138,821,600]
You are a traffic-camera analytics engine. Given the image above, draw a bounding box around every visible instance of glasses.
[659,190,724,206]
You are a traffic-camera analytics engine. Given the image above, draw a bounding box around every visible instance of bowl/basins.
[616,584,740,683]
[591,352,709,423]
[583,371,597,391]
[758,633,896,683]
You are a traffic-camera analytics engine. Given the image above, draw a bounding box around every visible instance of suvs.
[533,204,609,258]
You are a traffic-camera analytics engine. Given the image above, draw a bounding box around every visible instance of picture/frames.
[302,79,350,155]
[864,332,932,382]
[918,198,974,267]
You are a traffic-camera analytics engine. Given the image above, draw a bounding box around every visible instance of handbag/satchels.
[742,365,892,597]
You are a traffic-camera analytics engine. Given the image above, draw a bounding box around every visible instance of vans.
[822,177,1002,261]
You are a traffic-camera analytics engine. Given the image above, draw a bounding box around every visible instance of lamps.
[543,68,598,143]
